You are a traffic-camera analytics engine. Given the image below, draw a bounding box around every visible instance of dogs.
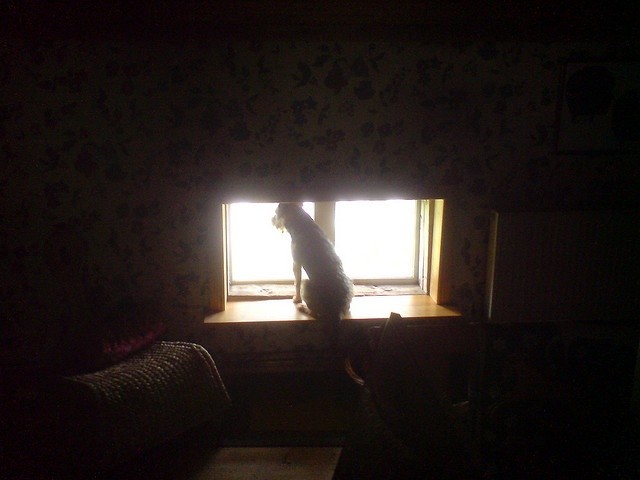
[272,201,365,386]
